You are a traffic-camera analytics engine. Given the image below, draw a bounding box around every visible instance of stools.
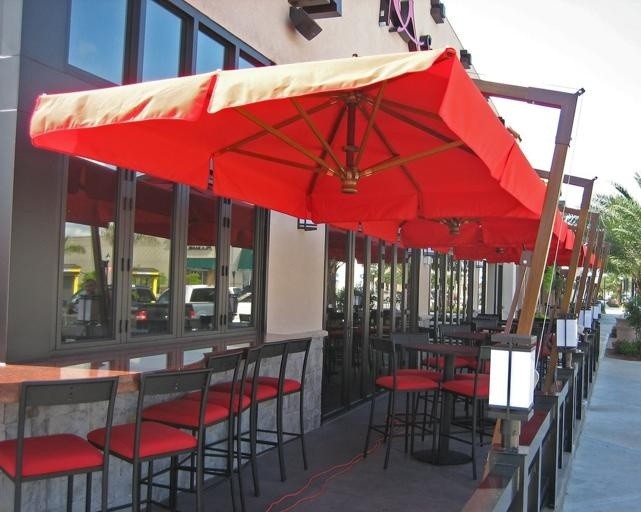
[237,336,313,480]
[0,376,118,512]
[174,347,264,512]
[142,351,245,512]
[87,368,212,512]
[210,341,285,497]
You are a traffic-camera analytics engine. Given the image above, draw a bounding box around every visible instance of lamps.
[555,301,602,368]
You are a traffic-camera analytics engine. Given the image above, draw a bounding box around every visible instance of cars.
[60,281,253,329]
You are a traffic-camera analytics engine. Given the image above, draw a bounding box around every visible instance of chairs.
[362,314,554,481]
